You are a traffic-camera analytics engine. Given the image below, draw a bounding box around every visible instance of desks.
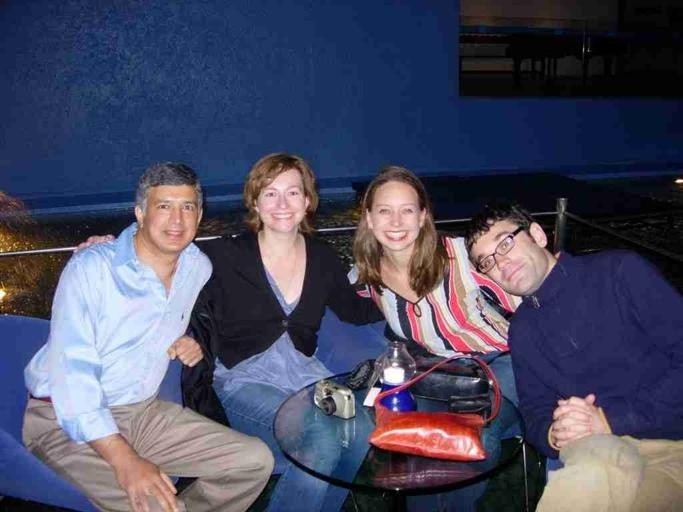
[273,372,533,511]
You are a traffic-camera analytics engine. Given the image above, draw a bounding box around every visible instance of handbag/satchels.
[408,359,490,403]
[368,355,501,462]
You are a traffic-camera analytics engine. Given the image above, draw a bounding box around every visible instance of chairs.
[1,310,100,512]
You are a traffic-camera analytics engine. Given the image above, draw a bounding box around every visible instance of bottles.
[374,342,418,424]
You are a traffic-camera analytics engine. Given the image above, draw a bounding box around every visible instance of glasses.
[476,226,526,274]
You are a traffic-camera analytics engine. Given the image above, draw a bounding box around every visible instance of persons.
[22,161,276,512]
[463,198,682,512]
[346,165,523,512]
[74,153,386,512]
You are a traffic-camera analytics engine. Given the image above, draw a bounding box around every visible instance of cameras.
[314,380,355,419]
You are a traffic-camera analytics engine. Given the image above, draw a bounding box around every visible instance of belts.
[29,393,52,403]
